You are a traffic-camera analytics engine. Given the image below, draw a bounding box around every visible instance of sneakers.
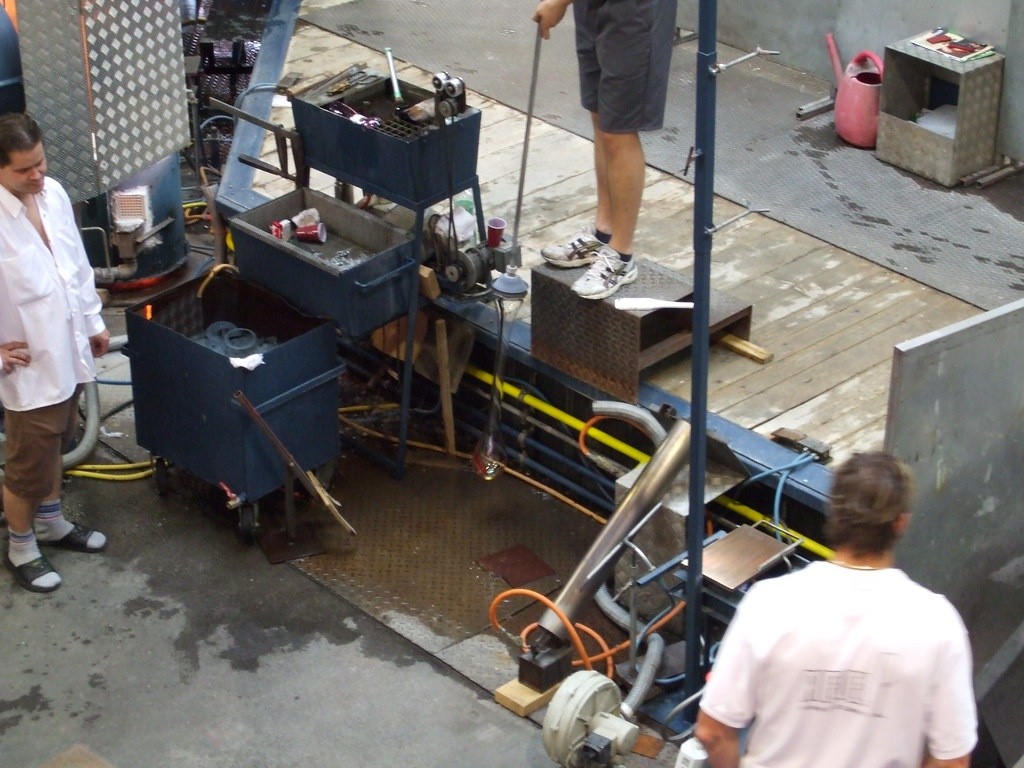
[540,222,640,302]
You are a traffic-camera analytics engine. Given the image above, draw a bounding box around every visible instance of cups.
[487,218,507,246]
[296,224,327,243]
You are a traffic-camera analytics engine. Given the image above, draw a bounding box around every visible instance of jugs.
[825,34,884,149]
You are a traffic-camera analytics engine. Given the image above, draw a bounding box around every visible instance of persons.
[0,110,111,593]
[531,0,680,302]
[692,443,979,768]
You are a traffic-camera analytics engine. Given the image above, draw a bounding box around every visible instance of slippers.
[35,521,108,554]
[2,553,63,594]
